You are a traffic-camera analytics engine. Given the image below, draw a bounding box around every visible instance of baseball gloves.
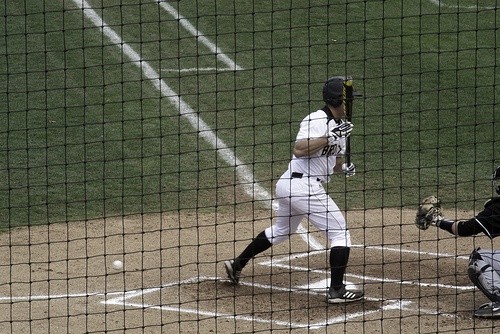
[414,194,446,230]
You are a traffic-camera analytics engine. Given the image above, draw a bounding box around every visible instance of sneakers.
[224,259,241,285]
[474,302,500,318]
[327,284,362,304]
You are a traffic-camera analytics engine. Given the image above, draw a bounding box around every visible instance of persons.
[416,165,500,319]
[224,76,362,303]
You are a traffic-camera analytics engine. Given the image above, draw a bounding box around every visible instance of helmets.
[322,76,346,109]
[491,166,500,196]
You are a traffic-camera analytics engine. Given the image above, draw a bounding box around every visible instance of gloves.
[342,162,356,177]
[325,122,354,146]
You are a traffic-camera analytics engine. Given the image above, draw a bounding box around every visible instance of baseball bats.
[341,76,355,168]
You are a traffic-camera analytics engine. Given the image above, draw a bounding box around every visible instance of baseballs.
[112,260,123,271]
